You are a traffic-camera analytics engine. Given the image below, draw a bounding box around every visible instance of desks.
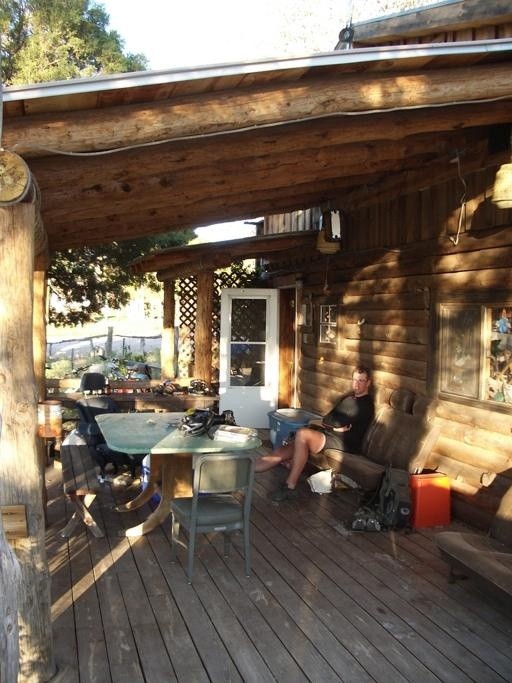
[103,391,222,411]
[89,410,264,540]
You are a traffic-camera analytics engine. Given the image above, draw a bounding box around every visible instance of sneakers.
[267,482,299,502]
[351,506,381,532]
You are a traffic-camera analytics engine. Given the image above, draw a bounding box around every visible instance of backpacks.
[375,468,413,531]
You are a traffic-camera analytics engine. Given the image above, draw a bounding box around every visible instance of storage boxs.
[268,405,323,450]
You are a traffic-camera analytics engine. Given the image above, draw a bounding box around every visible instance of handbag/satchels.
[193,410,236,426]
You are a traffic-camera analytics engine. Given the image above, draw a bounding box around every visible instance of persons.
[496,308,511,332]
[254,366,374,501]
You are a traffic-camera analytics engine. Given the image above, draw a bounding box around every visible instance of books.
[213,424,250,443]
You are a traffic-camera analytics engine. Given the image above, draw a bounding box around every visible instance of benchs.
[58,443,105,540]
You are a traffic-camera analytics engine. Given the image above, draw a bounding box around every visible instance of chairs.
[167,450,257,585]
[77,373,138,480]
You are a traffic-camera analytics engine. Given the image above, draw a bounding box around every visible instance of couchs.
[434,485,512,602]
[313,386,443,508]
